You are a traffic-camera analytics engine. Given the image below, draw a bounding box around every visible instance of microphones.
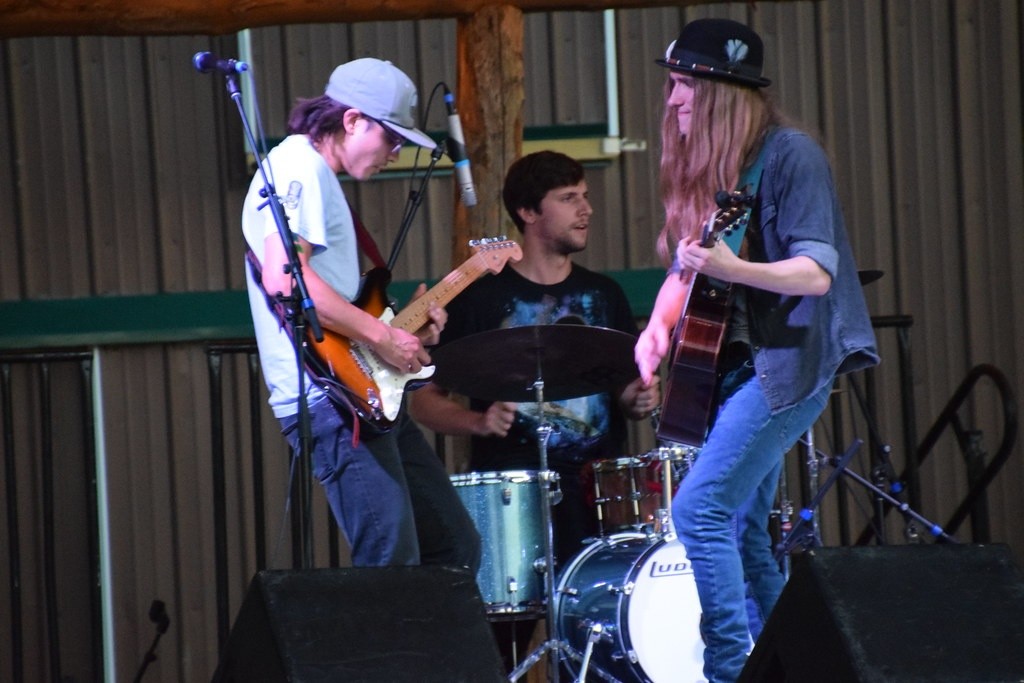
[193,51,248,74]
[442,91,478,208]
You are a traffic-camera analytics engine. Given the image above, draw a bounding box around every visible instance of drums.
[447,468,559,622]
[551,532,714,683]
[590,447,697,538]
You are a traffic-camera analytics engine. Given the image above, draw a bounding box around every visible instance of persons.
[239,56,483,581]
[404,148,661,682]
[634,15,881,683]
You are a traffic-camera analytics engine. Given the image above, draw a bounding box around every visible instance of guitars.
[292,233,523,434]
[655,189,752,448]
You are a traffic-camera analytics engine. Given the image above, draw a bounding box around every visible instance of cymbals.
[426,324,642,403]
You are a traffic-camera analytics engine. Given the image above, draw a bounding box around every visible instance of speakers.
[209,565,509,682]
[738,542,1024,683]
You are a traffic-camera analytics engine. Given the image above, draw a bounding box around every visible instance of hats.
[326,57,438,149]
[657,18,772,85]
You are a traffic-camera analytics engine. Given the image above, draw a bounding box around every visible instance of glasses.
[374,117,411,148]
